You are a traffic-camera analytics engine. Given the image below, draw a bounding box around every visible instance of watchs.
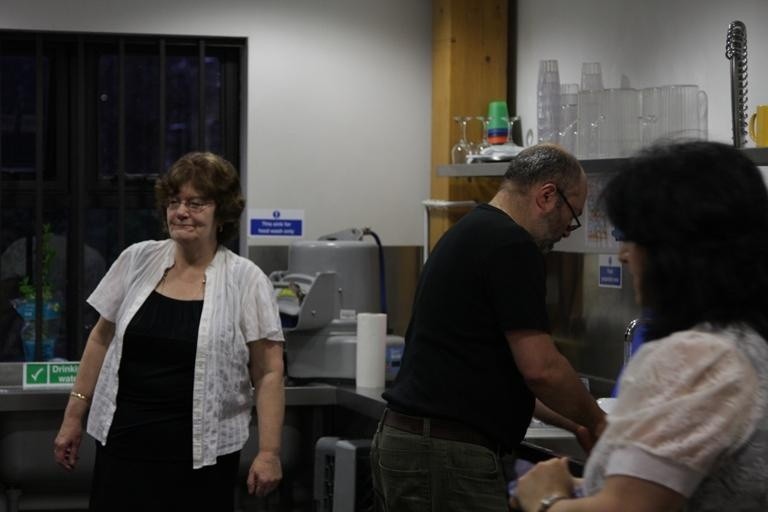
[538,494,571,512]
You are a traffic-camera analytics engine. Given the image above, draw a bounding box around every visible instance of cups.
[750,105,768,148]
[537,60,560,145]
[660,84,708,143]
[559,83,577,154]
[642,86,660,144]
[578,61,600,158]
[602,89,641,158]
[501,117,520,147]
[475,116,492,162]
[451,116,471,164]
[487,101,509,144]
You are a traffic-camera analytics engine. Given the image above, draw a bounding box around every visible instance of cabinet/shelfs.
[435,147,768,178]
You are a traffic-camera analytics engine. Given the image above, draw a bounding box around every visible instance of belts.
[383,411,498,452]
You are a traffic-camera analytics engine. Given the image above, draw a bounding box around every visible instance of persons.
[54,150,287,512]
[510,139,768,512]
[368,143,608,512]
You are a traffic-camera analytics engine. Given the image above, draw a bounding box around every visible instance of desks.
[338,383,610,479]
[1,358,337,512]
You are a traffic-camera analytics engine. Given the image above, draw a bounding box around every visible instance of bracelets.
[68,391,89,402]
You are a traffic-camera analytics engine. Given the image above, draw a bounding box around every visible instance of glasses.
[164,196,210,211]
[556,187,582,233]
